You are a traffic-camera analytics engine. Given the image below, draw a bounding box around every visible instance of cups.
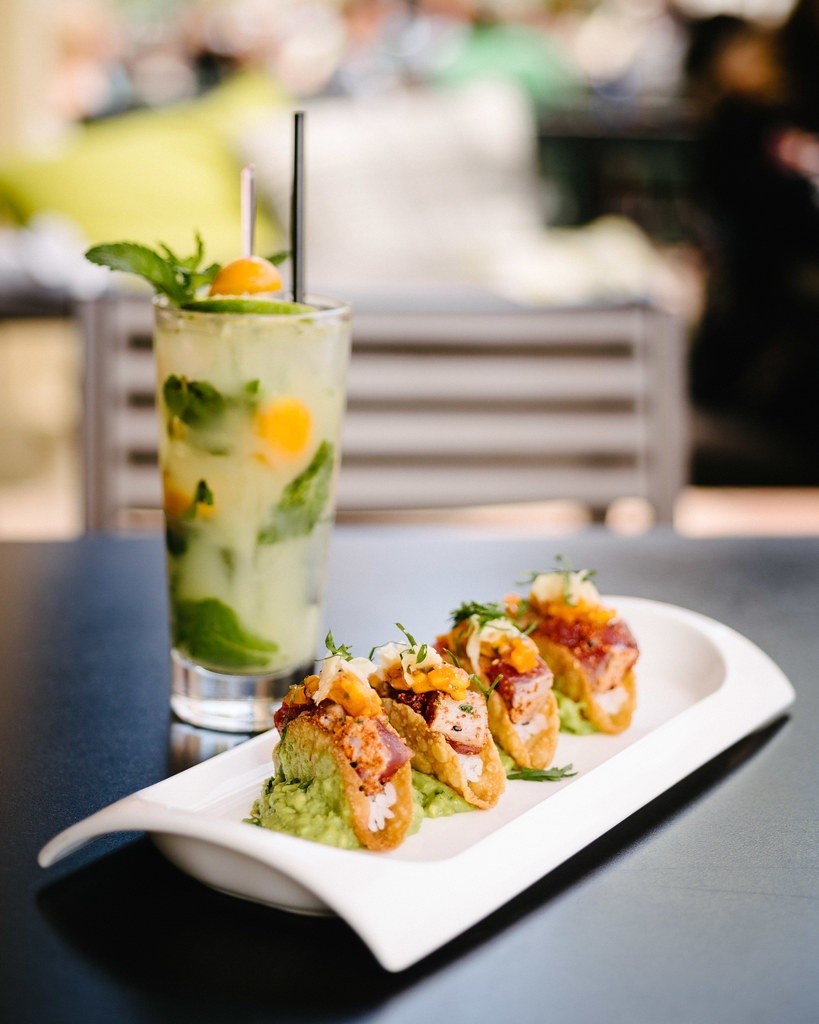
[152,303,352,735]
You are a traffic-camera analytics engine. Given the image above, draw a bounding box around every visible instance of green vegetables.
[85,231,350,673]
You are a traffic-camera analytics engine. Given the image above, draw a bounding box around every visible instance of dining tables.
[0,536,819,1024]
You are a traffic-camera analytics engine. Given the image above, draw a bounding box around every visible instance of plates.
[37,594,795,972]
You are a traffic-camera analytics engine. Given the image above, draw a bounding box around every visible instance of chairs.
[88,313,681,540]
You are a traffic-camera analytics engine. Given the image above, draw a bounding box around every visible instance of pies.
[253,556,638,851]
